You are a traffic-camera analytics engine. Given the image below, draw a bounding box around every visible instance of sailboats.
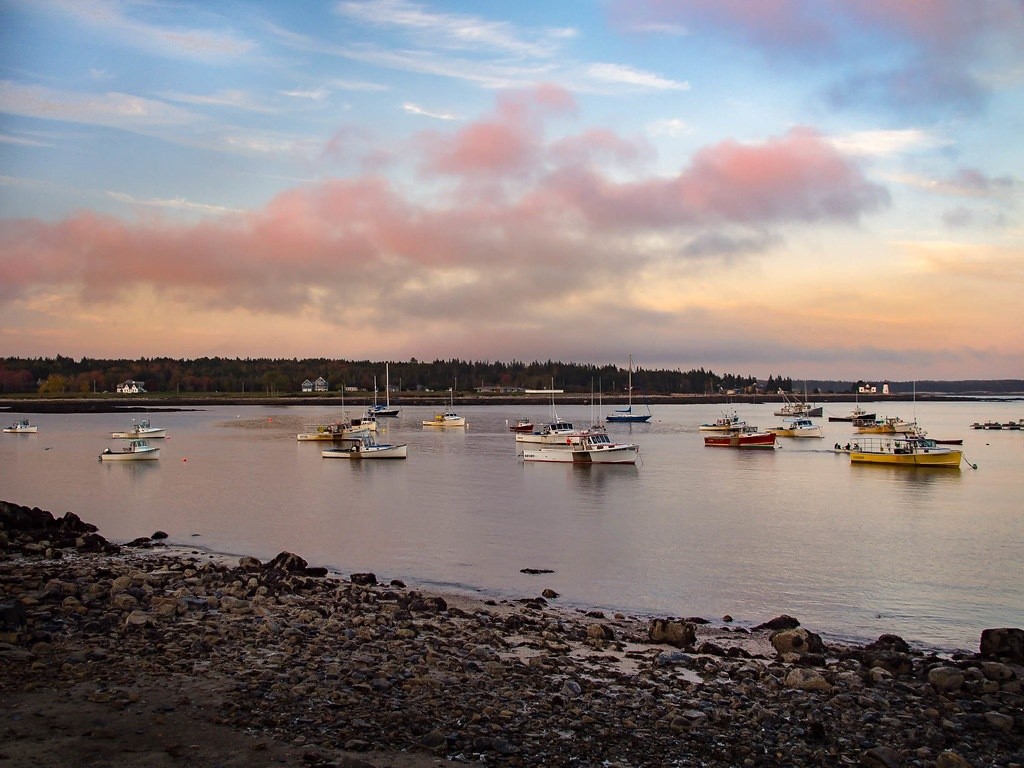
[606,354,654,423]
[366,362,400,416]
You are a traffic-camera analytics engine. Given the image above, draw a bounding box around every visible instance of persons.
[834,442,841,449]
[845,443,851,450]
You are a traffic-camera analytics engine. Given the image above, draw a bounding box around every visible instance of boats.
[832,447,859,454]
[113,417,167,439]
[321,434,408,460]
[2,418,38,434]
[515,376,609,445]
[295,420,372,440]
[328,408,378,431]
[698,400,759,434]
[849,434,963,467]
[829,388,877,423]
[98,437,162,461]
[764,413,826,439]
[510,416,534,433]
[773,380,823,417]
[421,388,466,427]
[704,430,778,449]
[857,416,917,434]
[522,374,640,463]
